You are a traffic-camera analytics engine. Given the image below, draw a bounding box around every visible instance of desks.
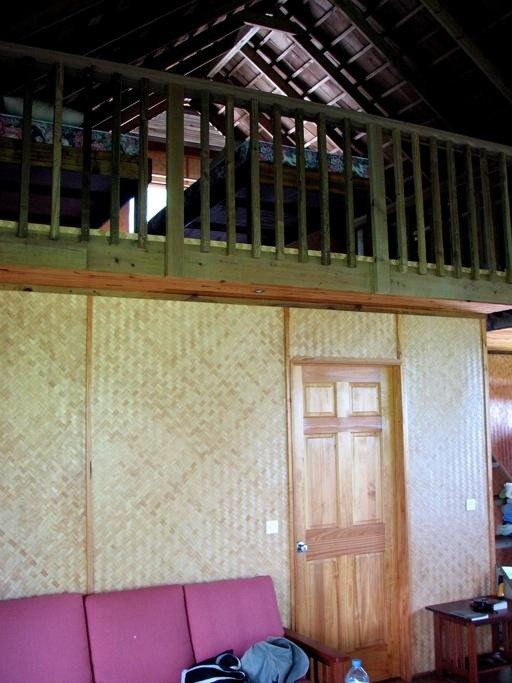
[425,596,512,683]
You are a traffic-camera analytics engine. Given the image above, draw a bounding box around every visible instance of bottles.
[343,657,369,683]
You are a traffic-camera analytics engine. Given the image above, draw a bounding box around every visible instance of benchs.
[283,627,350,683]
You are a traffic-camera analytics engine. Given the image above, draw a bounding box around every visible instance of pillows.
[1,574,283,683]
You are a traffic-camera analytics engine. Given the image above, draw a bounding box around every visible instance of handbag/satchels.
[182,648,247,683]
[240,636,309,683]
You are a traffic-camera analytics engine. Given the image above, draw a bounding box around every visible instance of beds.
[148,141,369,246]
[0,94,152,229]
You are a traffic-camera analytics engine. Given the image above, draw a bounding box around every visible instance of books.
[471,597,508,611]
[447,609,489,622]
